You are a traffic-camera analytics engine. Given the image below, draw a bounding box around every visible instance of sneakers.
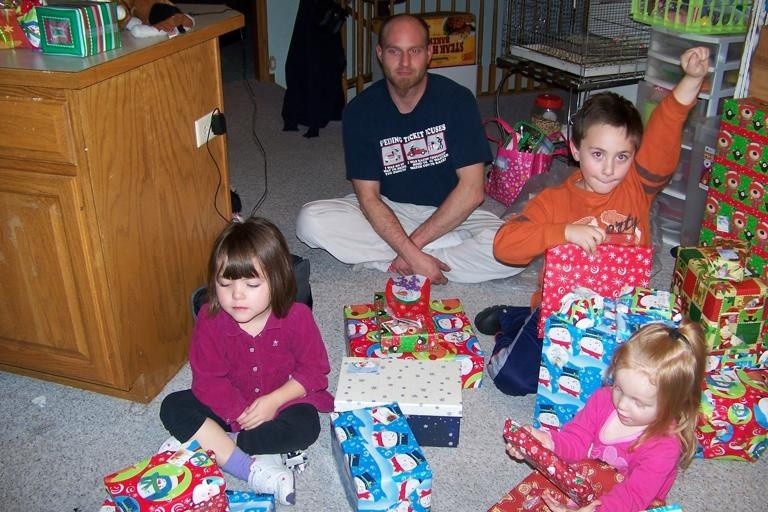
[158,436,182,455]
[475,304,531,335]
[246,454,296,507]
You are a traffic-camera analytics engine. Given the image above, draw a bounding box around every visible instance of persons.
[505,319,709,512]
[293,13,528,286]
[474,45,711,397]
[156,218,336,506]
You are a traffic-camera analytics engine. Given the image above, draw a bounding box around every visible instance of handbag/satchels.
[484,140,554,208]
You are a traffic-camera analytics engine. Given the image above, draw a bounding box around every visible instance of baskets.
[629,0,753,36]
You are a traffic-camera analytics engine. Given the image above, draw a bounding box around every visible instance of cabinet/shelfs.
[0,0,245,403]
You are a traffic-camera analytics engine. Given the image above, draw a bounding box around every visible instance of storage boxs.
[328,265,486,512]
[486,26,767,511]
[101,441,278,511]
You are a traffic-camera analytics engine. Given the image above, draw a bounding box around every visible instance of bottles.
[532,93,563,133]
